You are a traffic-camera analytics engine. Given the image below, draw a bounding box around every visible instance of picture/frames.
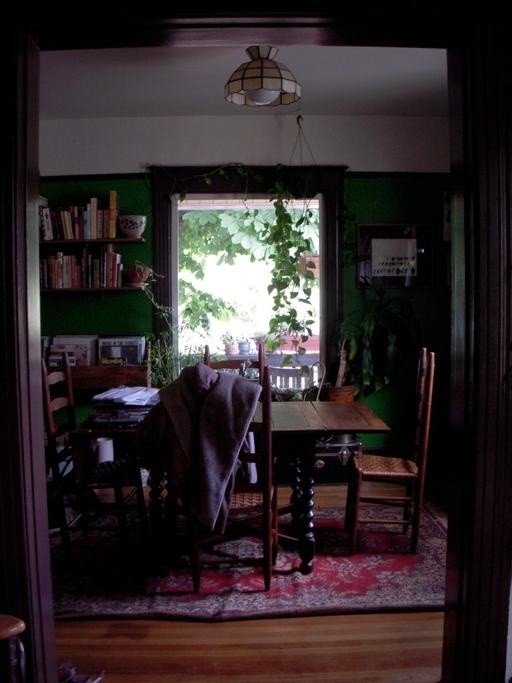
[356,222,418,257]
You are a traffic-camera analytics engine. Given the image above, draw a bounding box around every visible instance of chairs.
[183,358,279,593]
[344,348,435,554]
[38,352,150,556]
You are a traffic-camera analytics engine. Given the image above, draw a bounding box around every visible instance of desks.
[77,400,392,577]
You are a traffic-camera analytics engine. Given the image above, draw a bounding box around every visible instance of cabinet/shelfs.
[39,238,147,292]
[41,365,151,391]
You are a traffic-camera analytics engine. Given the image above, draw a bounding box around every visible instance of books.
[91,385,162,428]
[49,334,145,368]
[37,190,124,289]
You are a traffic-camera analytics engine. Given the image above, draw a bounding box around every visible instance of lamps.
[223,44,302,108]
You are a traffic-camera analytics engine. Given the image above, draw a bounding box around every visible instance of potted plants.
[118,207,153,238]
[339,300,395,450]
[116,247,165,288]
[258,116,323,369]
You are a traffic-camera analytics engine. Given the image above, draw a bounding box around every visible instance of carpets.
[49,501,447,622]
